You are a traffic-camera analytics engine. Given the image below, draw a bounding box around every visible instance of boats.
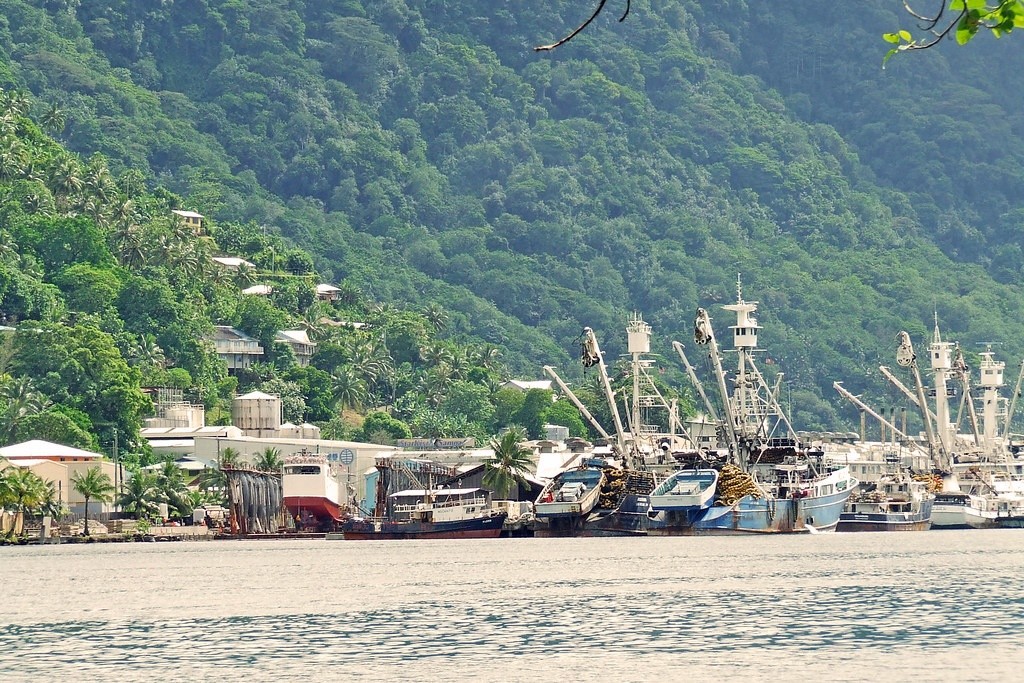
[835,473,936,532]
[931,491,1023,530]
[649,466,718,510]
[534,437,859,538]
[533,463,605,516]
[280,445,348,524]
[343,470,509,539]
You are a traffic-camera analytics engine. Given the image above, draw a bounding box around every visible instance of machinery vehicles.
[572,327,634,472]
[952,341,985,463]
[895,331,952,476]
[693,307,742,469]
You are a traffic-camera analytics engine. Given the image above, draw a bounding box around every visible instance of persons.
[206,515,212,529]
[5,529,14,546]
[295,514,302,530]
[170,514,178,522]
